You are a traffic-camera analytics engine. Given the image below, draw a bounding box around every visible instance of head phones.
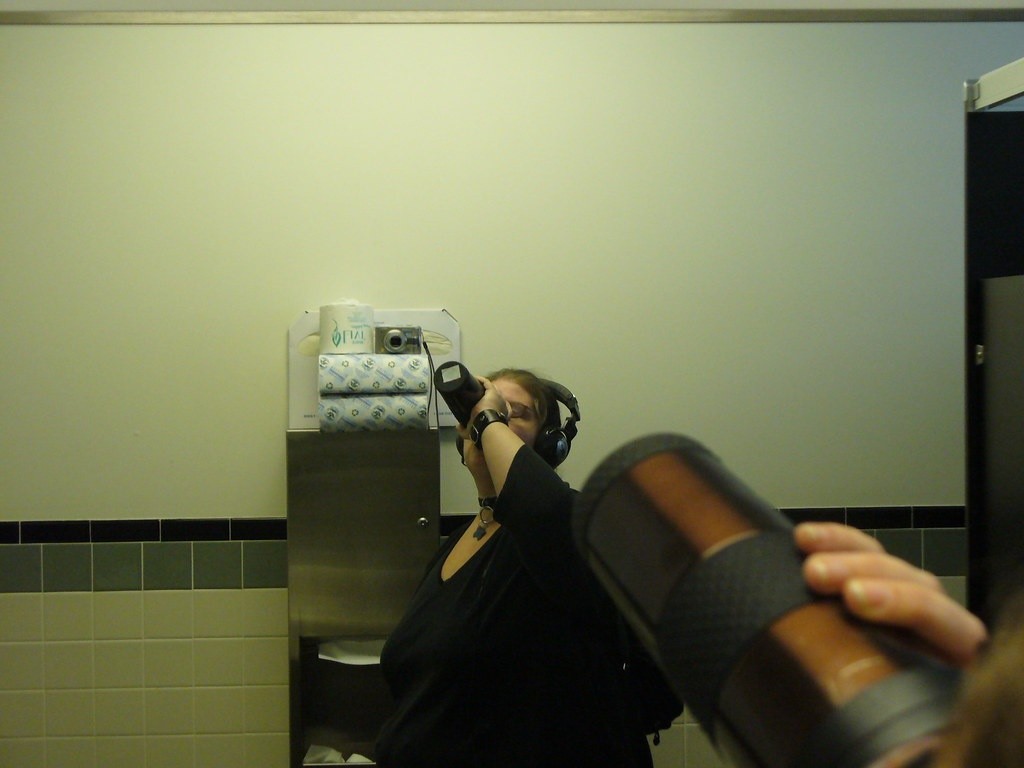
[456,378,581,470]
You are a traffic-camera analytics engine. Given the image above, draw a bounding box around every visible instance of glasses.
[508,400,535,419]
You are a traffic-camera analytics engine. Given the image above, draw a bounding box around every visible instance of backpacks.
[622,614,683,746]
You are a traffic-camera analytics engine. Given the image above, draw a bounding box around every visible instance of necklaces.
[473,495,497,540]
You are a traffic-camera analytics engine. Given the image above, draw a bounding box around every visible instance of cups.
[574,436,966,767]
[433,361,486,429]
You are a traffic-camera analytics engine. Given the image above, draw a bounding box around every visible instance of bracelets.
[469,409,508,448]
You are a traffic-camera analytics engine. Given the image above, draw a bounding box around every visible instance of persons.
[794,523,1024,768]
[369,368,686,768]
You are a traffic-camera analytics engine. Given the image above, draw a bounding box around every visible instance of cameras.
[374,327,422,355]
